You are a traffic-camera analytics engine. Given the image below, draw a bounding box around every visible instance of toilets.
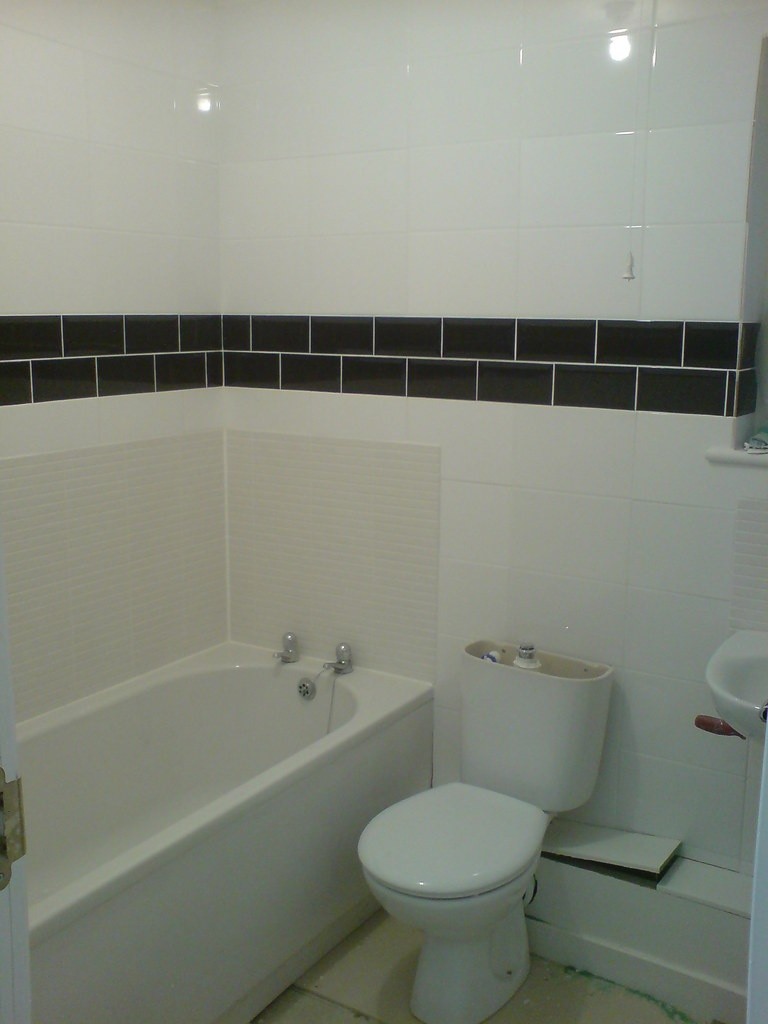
[353,638,617,1023]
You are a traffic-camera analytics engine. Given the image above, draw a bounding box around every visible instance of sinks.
[703,629,768,747]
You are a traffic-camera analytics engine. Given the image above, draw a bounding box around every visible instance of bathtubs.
[0,640,437,1023]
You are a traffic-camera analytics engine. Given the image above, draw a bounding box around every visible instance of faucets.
[270,631,299,665]
[321,642,355,676]
